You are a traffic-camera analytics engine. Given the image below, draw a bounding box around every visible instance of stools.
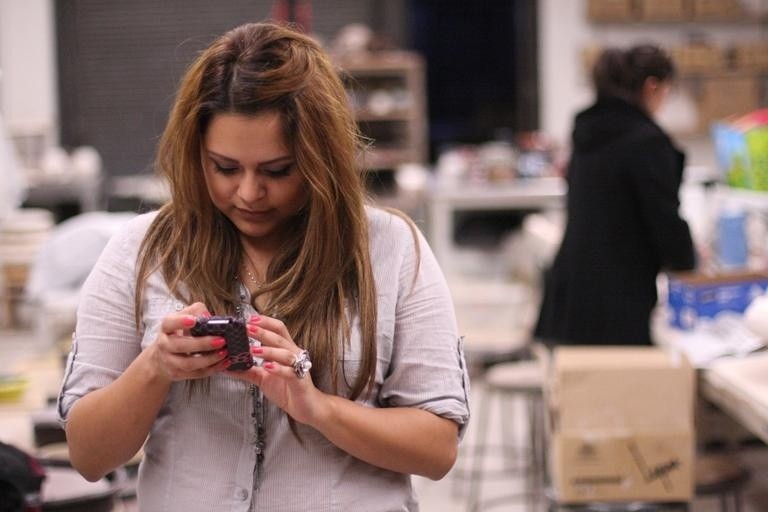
[453,319,548,512]
[693,453,752,512]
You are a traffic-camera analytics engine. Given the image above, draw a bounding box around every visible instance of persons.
[53,17,473,512]
[531,39,712,352]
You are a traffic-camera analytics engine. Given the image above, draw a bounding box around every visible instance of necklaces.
[239,249,264,289]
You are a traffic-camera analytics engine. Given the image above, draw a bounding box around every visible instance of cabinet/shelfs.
[325,51,429,234]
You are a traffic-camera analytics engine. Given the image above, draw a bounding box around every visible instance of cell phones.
[192,318,256,378]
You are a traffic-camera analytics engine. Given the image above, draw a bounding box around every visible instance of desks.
[421,164,720,357]
[519,212,766,512]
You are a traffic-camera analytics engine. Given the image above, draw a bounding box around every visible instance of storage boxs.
[526,340,695,501]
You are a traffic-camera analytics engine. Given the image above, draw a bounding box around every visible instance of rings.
[292,347,313,378]
